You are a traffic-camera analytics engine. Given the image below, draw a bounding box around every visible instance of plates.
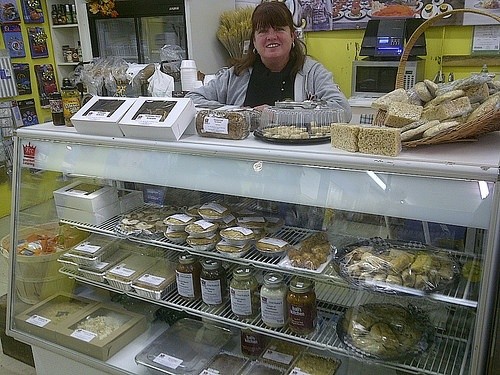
[253,123,345,145]
[333,238,463,295]
[336,303,437,360]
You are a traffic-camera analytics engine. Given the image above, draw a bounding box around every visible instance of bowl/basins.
[163,202,288,259]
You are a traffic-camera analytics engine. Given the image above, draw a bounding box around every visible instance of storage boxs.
[53,180,144,226]
[0,293,35,368]
[70,95,197,142]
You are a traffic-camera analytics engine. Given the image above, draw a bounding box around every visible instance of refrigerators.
[74,0,234,101]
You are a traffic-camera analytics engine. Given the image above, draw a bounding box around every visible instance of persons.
[184,2,352,128]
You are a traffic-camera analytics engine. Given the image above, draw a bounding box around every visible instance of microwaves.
[351,59,426,96]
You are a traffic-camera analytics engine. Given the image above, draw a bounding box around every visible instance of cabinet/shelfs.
[6,120,500,375]
[44,0,94,96]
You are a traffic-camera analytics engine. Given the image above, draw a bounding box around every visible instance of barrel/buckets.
[1,222,86,306]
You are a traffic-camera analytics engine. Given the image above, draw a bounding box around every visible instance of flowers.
[85,0,119,18]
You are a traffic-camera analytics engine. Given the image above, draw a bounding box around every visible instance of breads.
[330,72,500,157]
[343,304,426,359]
[345,245,454,289]
[425,0,449,16]
[287,231,332,270]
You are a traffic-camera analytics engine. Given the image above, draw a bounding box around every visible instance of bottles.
[49,94,65,125]
[72,49,79,62]
[61,85,82,127]
[61,5,65,25]
[66,48,74,63]
[51,4,57,26]
[77,41,83,62]
[65,5,72,25]
[62,45,70,64]
[57,4,62,25]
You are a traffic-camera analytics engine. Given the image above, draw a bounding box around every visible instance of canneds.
[175,253,318,335]
[61,86,82,127]
[49,93,65,125]
[66,48,79,62]
